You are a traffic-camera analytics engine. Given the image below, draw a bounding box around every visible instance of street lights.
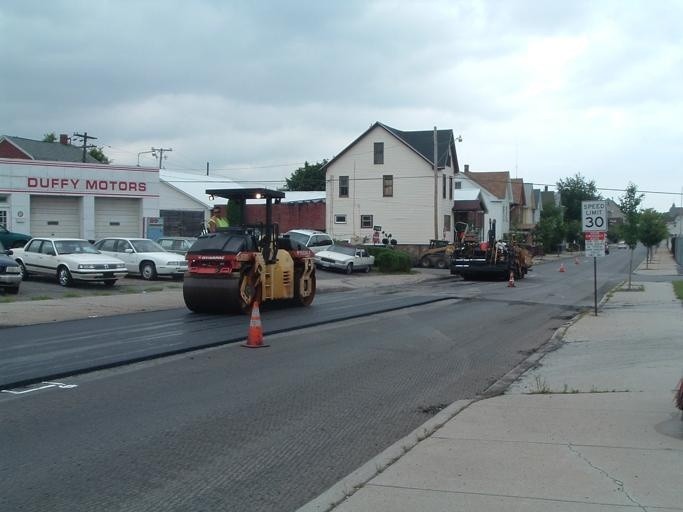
[434,127,462,238]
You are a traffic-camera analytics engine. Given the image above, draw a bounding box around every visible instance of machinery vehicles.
[418,218,531,281]
[183,188,316,313]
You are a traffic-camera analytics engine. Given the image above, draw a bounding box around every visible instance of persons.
[209,206,230,234]
[671,234,677,255]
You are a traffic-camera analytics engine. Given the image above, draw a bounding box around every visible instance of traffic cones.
[508,273,516,287]
[506,270,515,287]
[559,263,565,272]
[575,256,579,263]
[241,301,269,349]
[560,257,579,272]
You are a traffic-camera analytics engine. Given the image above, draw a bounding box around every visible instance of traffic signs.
[581,200,609,232]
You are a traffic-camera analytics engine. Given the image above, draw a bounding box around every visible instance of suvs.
[282,228,333,254]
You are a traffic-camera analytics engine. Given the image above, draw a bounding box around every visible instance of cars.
[76,238,188,280]
[314,244,374,273]
[156,237,197,254]
[10,237,128,287]
[618,241,627,249]
[0,224,33,293]
